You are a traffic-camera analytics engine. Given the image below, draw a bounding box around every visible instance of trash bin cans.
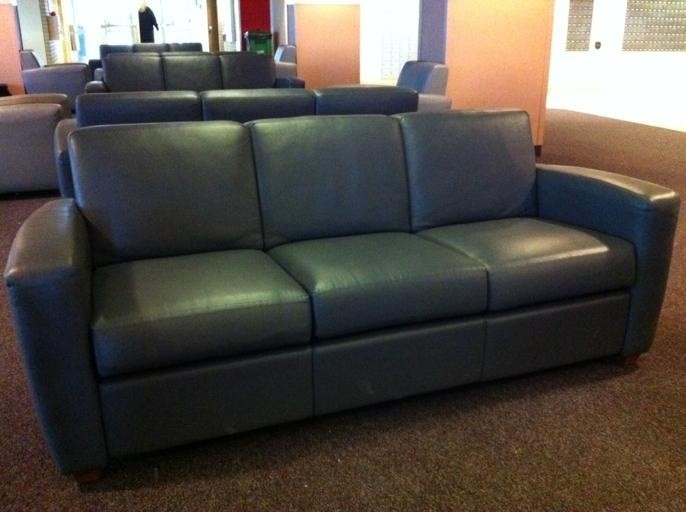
[245,31,273,55]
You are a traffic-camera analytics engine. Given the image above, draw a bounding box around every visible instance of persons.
[139,2,159,43]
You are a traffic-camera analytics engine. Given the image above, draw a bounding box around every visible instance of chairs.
[397,60,452,111]
[0,92,70,194]
[18,49,92,112]
[274,43,299,79]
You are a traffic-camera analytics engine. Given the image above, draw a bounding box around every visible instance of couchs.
[94,51,258,80]
[99,42,203,59]
[52,83,419,199]
[2,110,682,483]
[85,55,305,92]
